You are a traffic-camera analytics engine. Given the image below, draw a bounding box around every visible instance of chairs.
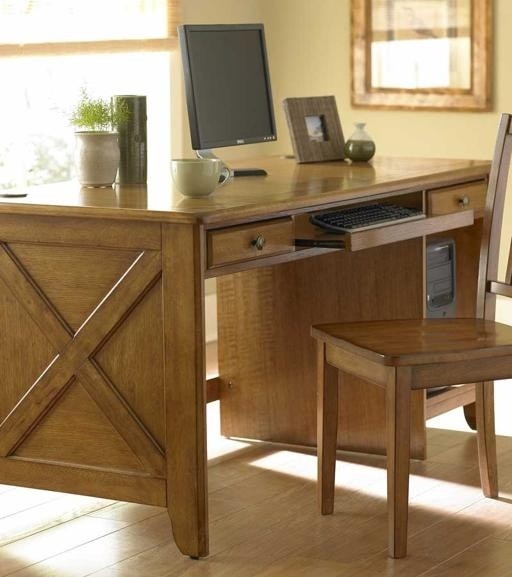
[311,112,511,560]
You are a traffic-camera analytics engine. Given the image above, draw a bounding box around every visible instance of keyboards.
[307,199,426,235]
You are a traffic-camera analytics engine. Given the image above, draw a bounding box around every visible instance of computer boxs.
[426,236,457,394]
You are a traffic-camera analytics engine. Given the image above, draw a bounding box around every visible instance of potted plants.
[76,94,128,186]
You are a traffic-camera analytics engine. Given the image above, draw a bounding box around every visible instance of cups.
[169,156,231,198]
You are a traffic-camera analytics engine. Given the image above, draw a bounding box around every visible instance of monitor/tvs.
[176,23,278,183]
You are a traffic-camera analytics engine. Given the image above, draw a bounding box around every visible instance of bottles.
[343,120,377,163]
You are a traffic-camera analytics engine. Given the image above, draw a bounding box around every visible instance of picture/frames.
[282,94,347,164]
[350,1,493,113]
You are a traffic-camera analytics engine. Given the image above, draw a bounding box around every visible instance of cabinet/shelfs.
[0,150,495,560]
